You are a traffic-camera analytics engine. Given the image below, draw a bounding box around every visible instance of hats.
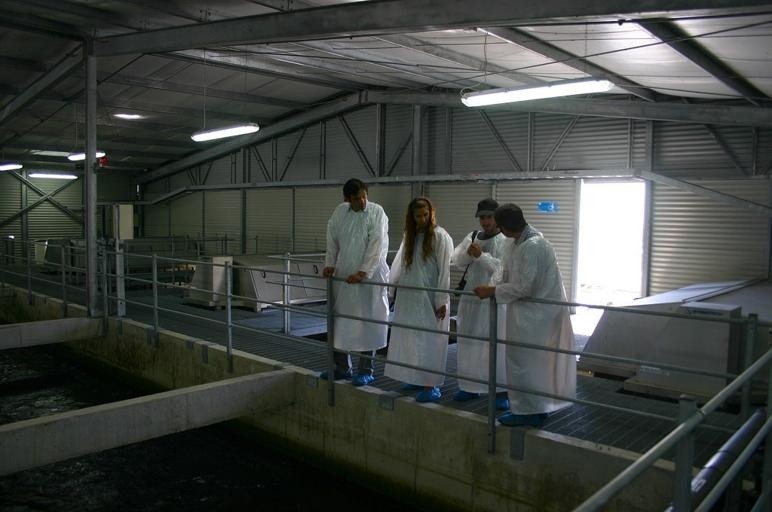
[476,199,499,217]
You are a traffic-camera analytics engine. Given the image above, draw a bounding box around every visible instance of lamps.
[459,21,613,108]
[189,46,261,143]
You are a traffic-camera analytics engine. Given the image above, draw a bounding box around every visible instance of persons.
[317,175,392,386]
[383,196,457,404]
[445,196,516,412]
[471,202,578,431]
